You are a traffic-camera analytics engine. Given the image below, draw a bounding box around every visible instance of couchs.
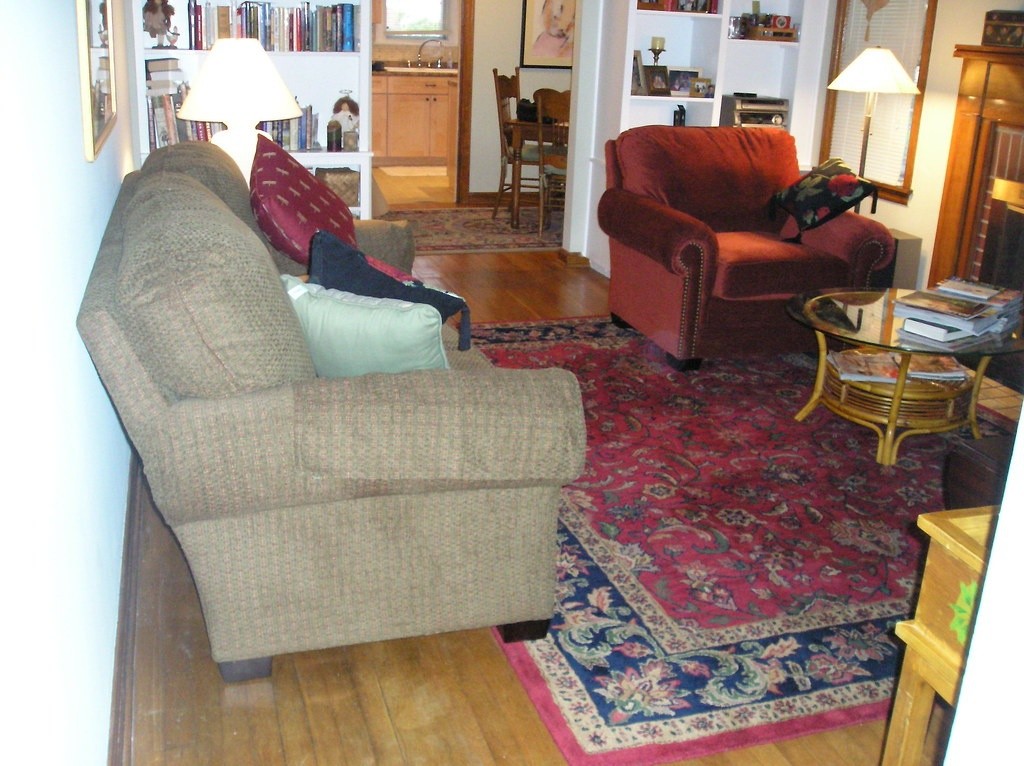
[76,141,586,683]
[598,127,895,370]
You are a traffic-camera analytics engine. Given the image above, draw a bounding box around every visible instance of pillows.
[306,229,471,351]
[249,133,357,265]
[280,274,452,379]
[766,157,878,245]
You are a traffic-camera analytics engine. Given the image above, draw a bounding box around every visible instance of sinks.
[384,67,457,72]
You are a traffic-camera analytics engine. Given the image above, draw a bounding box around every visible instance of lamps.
[827,47,921,213]
[176,38,303,188]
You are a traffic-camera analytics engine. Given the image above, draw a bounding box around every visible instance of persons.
[142,0,175,38]
[332,96,359,138]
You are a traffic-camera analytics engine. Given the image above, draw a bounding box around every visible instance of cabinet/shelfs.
[125,0,372,221]
[372,76,457,167]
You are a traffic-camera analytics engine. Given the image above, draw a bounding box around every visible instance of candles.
[651,36,665,49]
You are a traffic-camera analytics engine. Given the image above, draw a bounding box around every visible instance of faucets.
[417,39,442,65]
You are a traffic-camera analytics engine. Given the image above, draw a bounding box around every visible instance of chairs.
[533,88,571,239]
[491,67,551,226]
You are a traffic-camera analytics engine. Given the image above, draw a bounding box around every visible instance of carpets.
[373,205,564,256]
[457,317,1018,766]
[378,164,447,177]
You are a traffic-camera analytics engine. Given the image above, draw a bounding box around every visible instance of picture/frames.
[643,66,671,96]
[631,50,648,96]
[519,0,576,69]
[667,66,703,96]
[689,78,711,97]
[76,0,119,162]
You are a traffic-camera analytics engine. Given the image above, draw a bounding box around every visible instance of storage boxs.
[887,228,922,290]
[981,10,1024,48]
[316,168,358,208]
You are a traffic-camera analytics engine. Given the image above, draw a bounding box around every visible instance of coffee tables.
[784,287,1024,467]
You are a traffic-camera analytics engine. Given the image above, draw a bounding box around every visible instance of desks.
[506,118,570,229]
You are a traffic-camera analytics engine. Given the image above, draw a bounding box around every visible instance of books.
[892,275,1022,352]
[189,0,361,52]
[96,56,110,95]
[829,350,912,383]
[894,352,967,380]
[145,58,321,155]
